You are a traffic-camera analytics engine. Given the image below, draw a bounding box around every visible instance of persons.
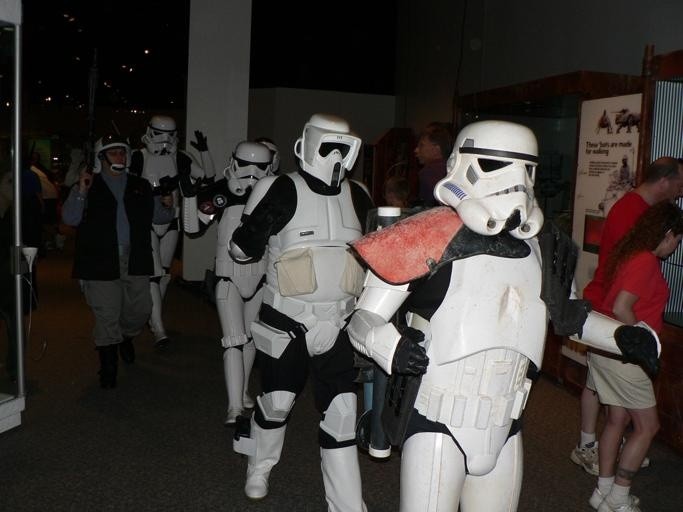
[347,121,662,512]
[228,114,377,512]
[128,116,215,351]
[569,156,683,475]
[179,141,273,424]
[385,121,458,209]
[23,134,155,389]
[587,202,683,511]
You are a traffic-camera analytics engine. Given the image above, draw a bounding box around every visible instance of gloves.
[174,175,195,197]
[615,324,663,377]
[390,325,429,378]
[189,129,208,151]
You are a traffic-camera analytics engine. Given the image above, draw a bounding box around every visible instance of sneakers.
[614,434,651,470]
[588,487,642,512]
[567,439,601,477]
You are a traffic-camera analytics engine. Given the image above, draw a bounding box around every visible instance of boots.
[118,334,136,365]
[243,406,287,502]
[319,444,369,512]
[147,273,172,348]
[221,339,257,428]
[93,344,120,392]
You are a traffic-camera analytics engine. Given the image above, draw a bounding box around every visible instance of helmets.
[292,112,362,190]
[140,115,180,157]
[91,133,133,175]
[222,139,280,197]
[431,118,546,241]
